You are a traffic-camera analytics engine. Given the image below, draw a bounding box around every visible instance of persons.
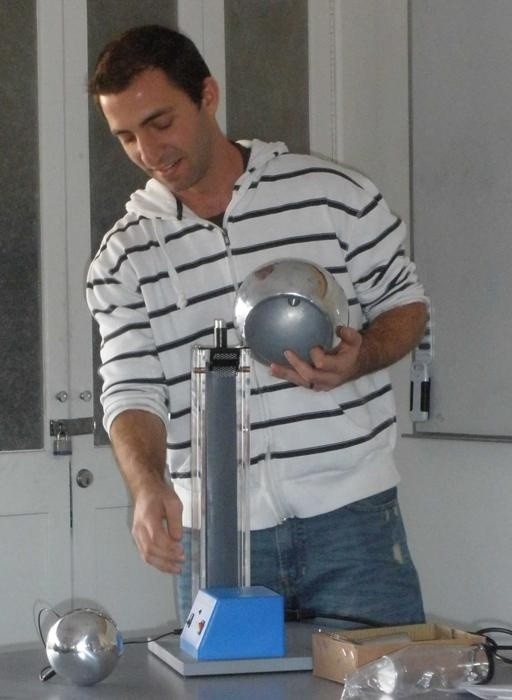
[83,25,433,630]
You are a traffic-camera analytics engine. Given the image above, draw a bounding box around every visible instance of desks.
[0,621,511,700]
